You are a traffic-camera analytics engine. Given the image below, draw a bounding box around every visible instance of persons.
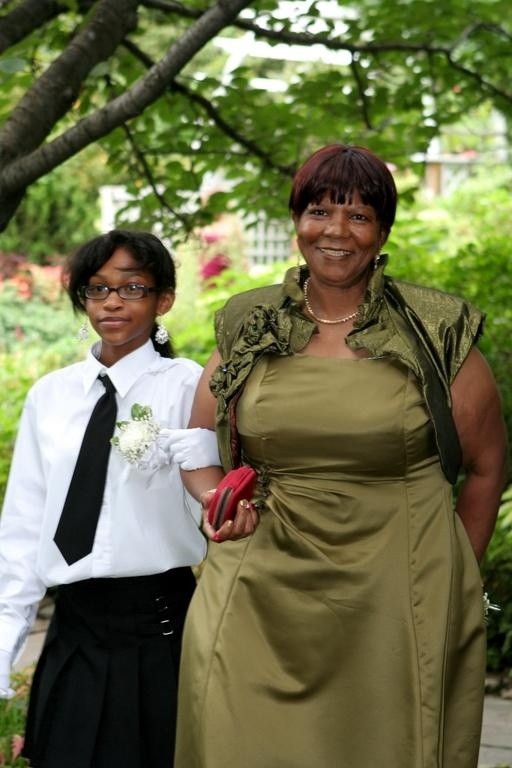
[174,142,508,768]
[1,231,226,767]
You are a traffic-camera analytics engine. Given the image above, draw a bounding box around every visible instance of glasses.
[80,282,162,301]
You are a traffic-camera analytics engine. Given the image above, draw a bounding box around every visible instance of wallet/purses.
[206,461,259,531]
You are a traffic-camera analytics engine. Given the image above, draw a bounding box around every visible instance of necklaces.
[302,274,358,325]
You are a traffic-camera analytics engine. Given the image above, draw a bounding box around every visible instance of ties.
[52,372,119,566]
